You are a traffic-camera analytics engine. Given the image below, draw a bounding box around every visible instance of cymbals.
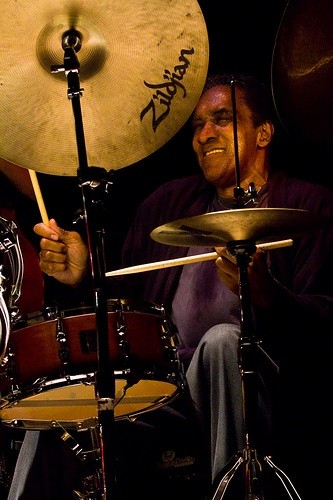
[0,300,188,432]
[0,0,209,181]
[149,208,312,246]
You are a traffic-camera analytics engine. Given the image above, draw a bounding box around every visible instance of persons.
[32,71,332,500]
